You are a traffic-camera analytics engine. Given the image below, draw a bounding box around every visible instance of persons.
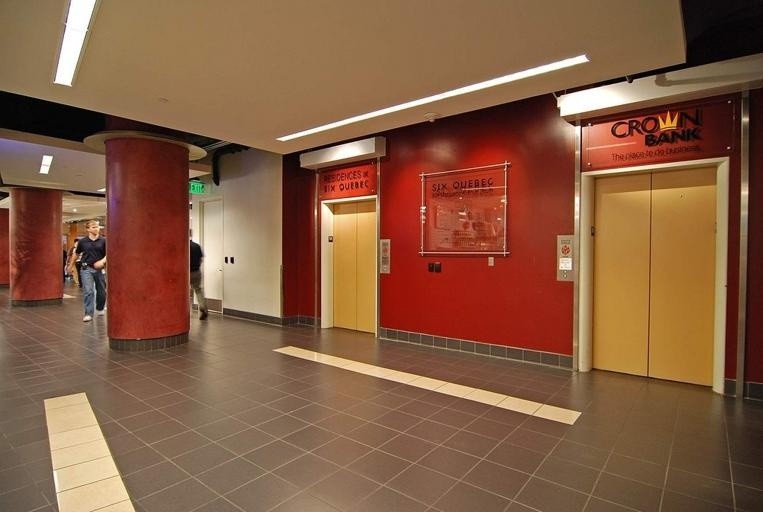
[64,219,106,322]
[189,229,208,322]
[61,237,108,291]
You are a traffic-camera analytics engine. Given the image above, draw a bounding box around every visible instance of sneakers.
[96,310,105,317]
[81,315,93,323]
[198,305,209,321]
